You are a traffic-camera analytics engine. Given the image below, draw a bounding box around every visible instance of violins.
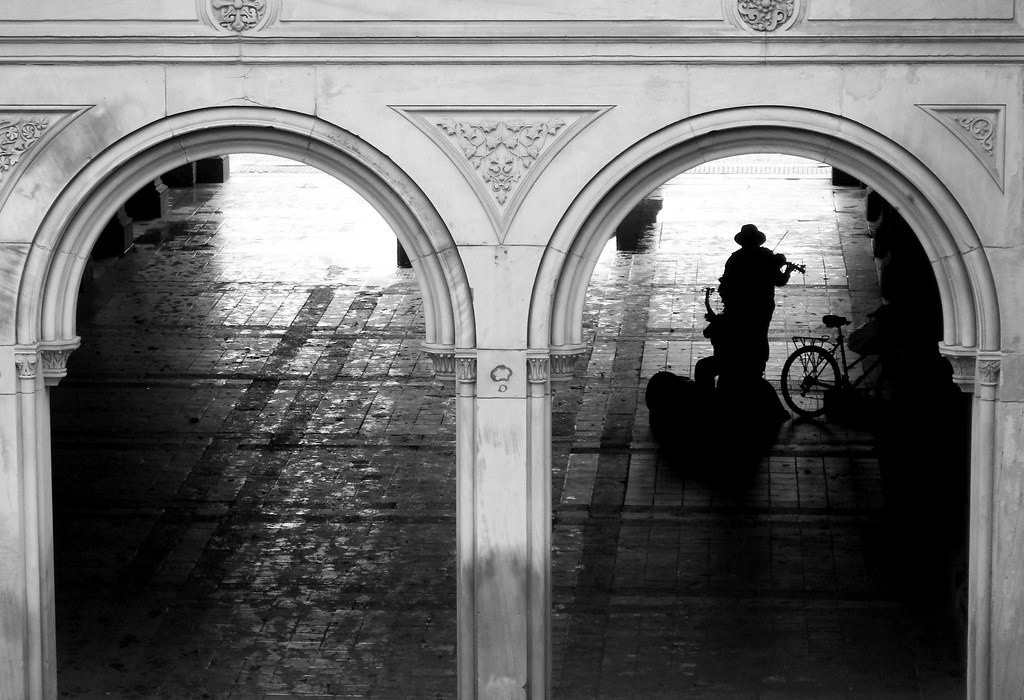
[761,248,810,275]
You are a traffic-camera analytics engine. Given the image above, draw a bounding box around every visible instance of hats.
[734,224,766,246]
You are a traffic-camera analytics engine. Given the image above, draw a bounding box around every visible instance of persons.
[693,224,795,391]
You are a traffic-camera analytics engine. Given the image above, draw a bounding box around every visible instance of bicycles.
[781,314,884,420]
